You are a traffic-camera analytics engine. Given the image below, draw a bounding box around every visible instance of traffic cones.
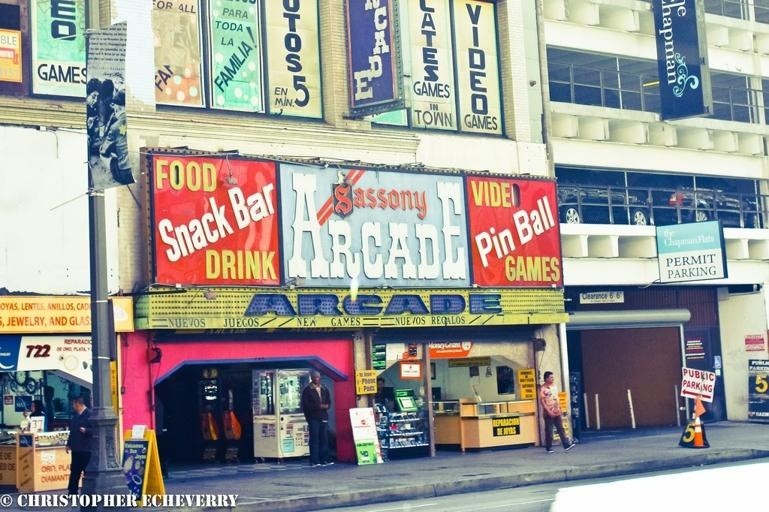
[691,399,705,448]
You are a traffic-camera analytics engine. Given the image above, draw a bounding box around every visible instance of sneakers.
[322,461,334,467]
[311,462,321,468]
[564,444,575,451]
[545,449,554,453]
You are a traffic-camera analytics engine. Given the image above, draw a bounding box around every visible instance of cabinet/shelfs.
[0,430,83,492]
[377,402,538,452]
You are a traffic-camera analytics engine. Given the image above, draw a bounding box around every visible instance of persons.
[539,369,575,454]
[301,369,335,468]
[376,378,393,401]
[87,72,134,186]
[46,386,57,418]
[64,395,91,497]
[23,399,45,422]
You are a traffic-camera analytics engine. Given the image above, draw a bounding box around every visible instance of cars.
[638,178,769,228]
[552,181,649,228]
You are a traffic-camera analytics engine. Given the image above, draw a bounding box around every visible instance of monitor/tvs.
[381,387,393,400]
[496,365,514,395]
[204,385,218,401]
[432,387,441,400]
[469,366,479,377]
